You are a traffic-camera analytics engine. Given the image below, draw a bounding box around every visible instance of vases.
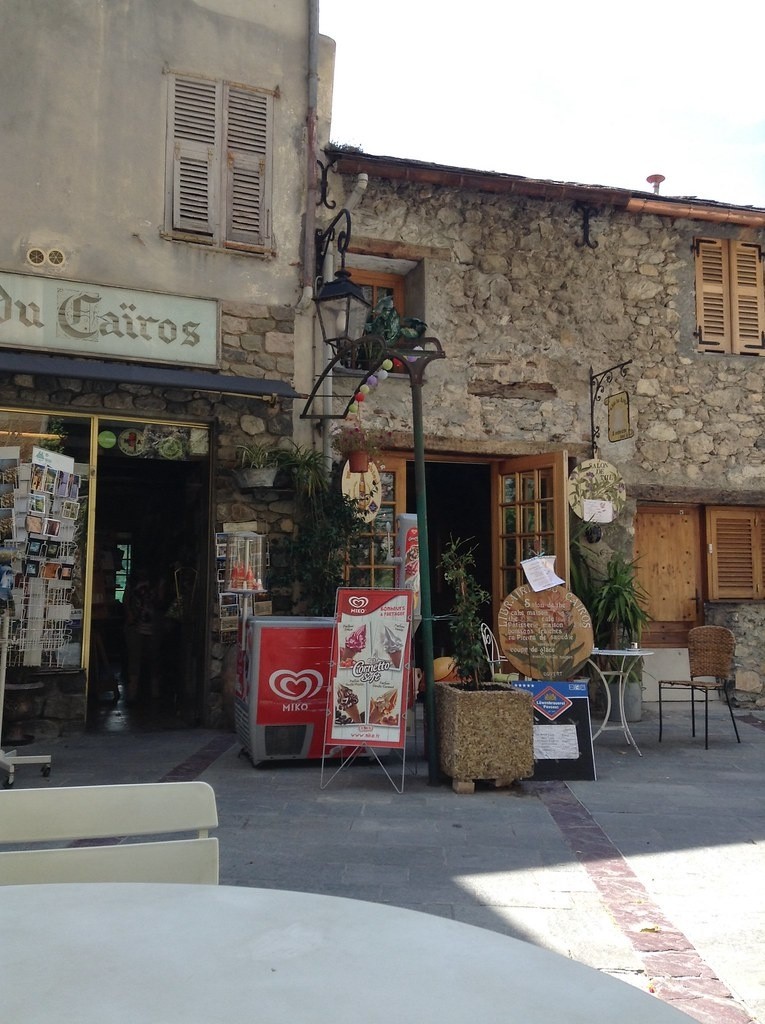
[349,451,368,473]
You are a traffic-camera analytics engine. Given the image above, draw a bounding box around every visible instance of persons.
[120,557,169,704]
[68,474,73,496]
[35,467,41,490]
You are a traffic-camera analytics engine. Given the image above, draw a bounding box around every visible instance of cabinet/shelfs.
[213,531,268,649]
[0,445,84,790]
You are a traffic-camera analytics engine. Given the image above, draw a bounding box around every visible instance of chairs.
[658,625,740,749]
[481,623,533,682]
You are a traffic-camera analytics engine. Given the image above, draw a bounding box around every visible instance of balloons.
[350,345,423,413]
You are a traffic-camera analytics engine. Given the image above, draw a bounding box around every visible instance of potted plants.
[229,442,329,498]
[433,534,535,793]
[591,551,657,722]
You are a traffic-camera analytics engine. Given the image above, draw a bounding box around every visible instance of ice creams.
[336,682,362,723]
[341,624,367,663]
[383,625,402,668]
[368,689,398,723]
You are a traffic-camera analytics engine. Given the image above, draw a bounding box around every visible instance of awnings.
[0,353,310,400]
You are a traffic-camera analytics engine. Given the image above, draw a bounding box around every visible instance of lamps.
[313,206,372,353]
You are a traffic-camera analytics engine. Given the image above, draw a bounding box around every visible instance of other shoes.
[125,700,136,707]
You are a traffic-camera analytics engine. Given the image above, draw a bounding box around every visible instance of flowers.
[331,425,393,467]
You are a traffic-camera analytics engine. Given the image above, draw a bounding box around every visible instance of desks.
[588,649,655,757]
